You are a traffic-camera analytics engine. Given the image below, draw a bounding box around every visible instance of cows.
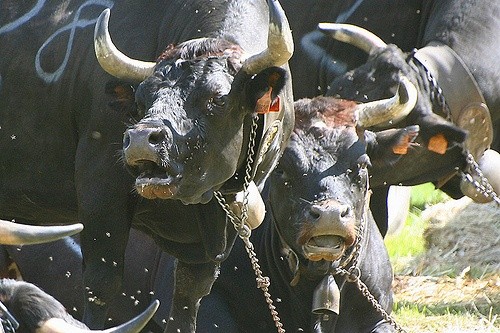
[0,0,500,333]
